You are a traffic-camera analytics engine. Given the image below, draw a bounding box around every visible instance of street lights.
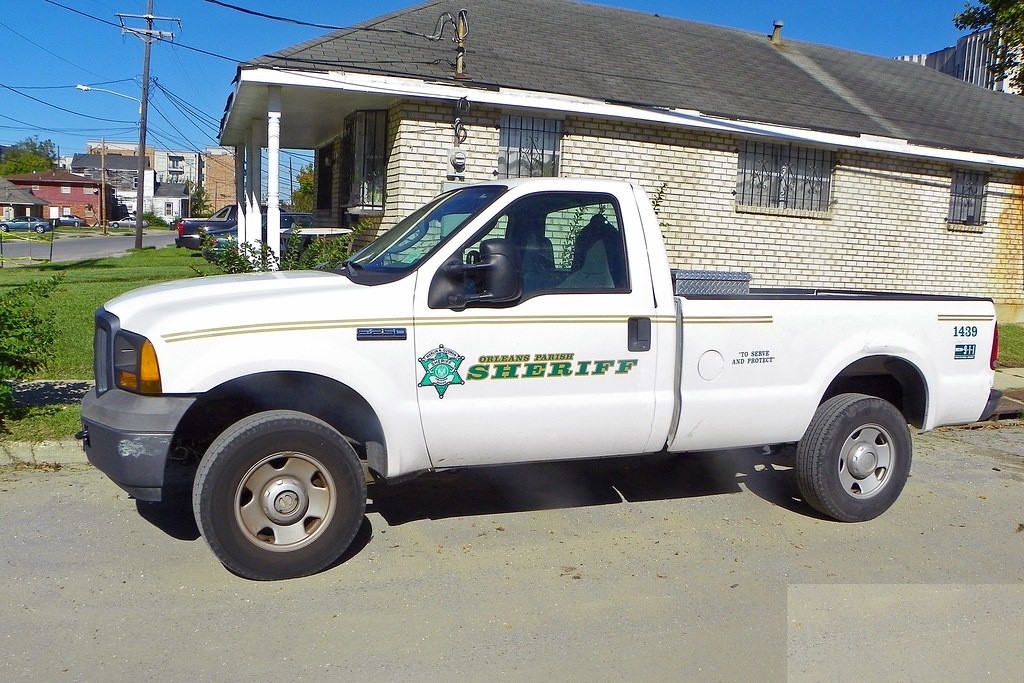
[75,83,148,249]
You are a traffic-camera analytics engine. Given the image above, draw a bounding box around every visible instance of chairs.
[519,222,621,290]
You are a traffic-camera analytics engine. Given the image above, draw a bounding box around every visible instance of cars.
[174,203,314,264]
[0,216,53,234]
[48,214,86,227]
[108,216,149,229]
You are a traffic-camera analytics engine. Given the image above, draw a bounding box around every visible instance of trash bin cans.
[170,222,175,231]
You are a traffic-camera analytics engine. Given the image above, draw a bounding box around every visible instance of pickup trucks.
[74,172,1005,582]
